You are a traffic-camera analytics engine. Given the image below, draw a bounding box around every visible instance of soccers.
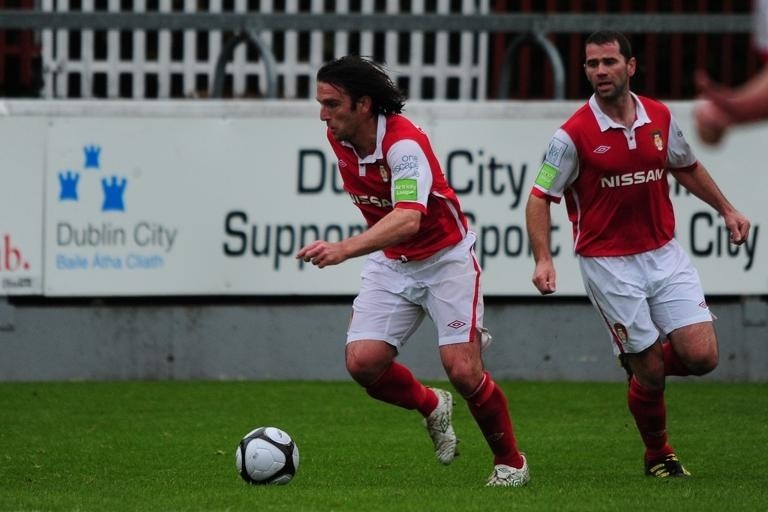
[231,426,302,486]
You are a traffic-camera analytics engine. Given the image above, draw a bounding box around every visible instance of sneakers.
[486,452,530,488]
[618,354,632,383]
[426,387,457,465]
[644,446,690,478]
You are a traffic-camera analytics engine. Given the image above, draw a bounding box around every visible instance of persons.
[523,27,753,482]
[294,53,533,490]
[693,65,768,150]
